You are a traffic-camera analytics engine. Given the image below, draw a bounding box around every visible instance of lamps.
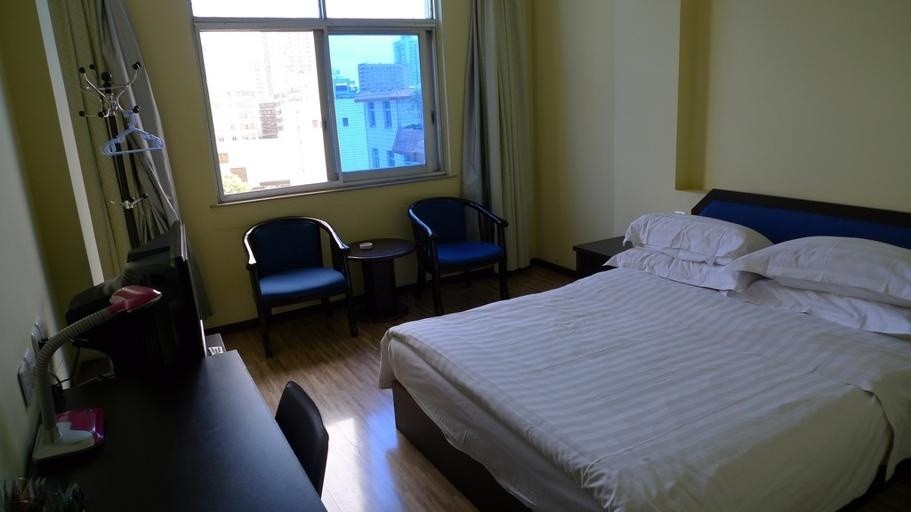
[29,286,164,464]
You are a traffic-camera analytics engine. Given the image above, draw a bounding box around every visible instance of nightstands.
[572,235,634,279]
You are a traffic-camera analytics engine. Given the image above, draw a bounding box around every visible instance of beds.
[391,187,911,511]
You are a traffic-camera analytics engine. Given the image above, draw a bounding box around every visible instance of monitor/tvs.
[65,220,206,376]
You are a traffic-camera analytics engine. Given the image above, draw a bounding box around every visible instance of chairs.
[408,196,510,315]
[274,380,329,499]
[244,215,359,358]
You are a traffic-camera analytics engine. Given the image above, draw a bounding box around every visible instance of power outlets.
[15,316,49,408]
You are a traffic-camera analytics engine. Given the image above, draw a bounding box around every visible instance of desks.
[16,348,328,512]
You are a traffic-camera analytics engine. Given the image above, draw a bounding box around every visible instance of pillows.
[622,214,772,266]
[726,279,911,340]
[602,246,766,294]
[721,235,910,309]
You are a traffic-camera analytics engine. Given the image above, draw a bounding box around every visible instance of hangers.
[100,109,165,157]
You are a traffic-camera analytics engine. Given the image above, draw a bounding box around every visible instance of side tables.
[337,239,415,324]
